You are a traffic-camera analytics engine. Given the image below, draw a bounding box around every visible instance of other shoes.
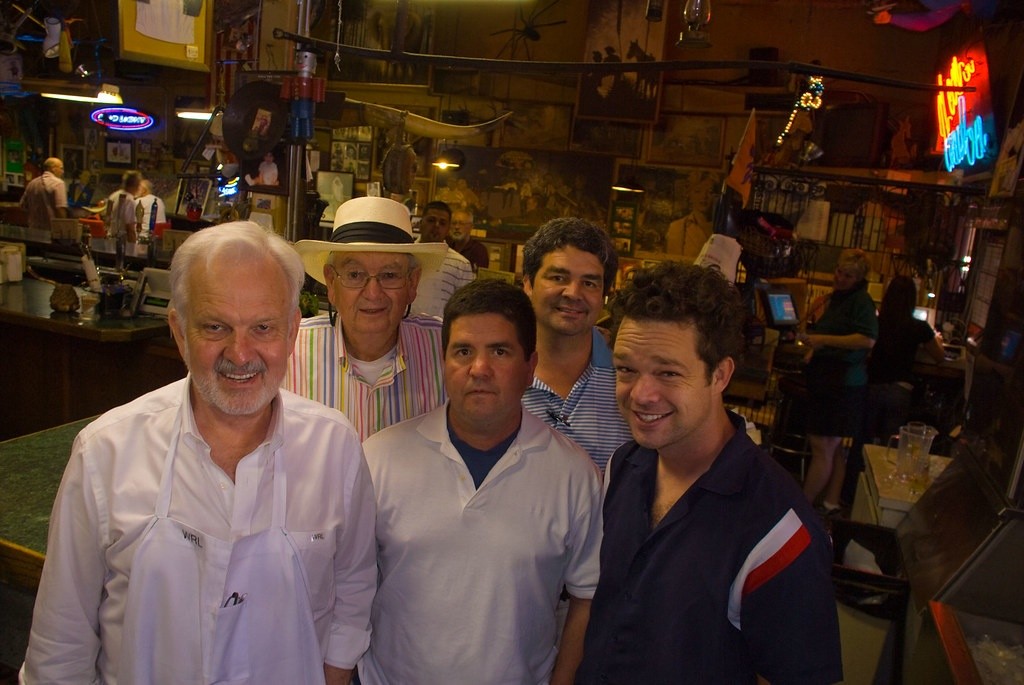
[813,496,842,515]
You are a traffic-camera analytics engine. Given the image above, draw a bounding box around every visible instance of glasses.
[328,264,410,290]
[53,165,63,170]
[450,221,471,227]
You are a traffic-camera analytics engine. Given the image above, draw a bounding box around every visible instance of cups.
[80,292,98,321]
[886,421,938,485]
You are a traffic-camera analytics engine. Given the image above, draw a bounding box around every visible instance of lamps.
[677,1,711,49]
[612,168,645,193]
[43,16,65,59]
[18,79,124,104]
[431,136,459,169]
[175,107,218,121]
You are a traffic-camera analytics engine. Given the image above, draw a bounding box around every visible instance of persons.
[523,216,634,476]
[566,260,843,685]
[21,158,68,228]
[876,277,944,442]
[353,280,607,685]
[668,171,721,254]
[244,153,278,186]
[409,202,472,315]
[450,210,488,269]
[18,220,377,685]
[110,172,140,243]
[279,195,447,441]
[136,181,167,233]
[791,248,879,510]
[279,42,325,141]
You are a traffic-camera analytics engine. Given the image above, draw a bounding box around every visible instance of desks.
[0,277,170,444]
[850,444,953,530]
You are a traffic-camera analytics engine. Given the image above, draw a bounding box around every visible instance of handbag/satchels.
[777,341,815,367]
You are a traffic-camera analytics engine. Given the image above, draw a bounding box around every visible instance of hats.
[294,197,448,286]
[692,233,742,288]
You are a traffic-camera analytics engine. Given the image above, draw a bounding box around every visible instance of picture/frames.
[328,125,373,182]
[105,136,135,168]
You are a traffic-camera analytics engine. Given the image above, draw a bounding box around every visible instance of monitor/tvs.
[761,288,801,329]
